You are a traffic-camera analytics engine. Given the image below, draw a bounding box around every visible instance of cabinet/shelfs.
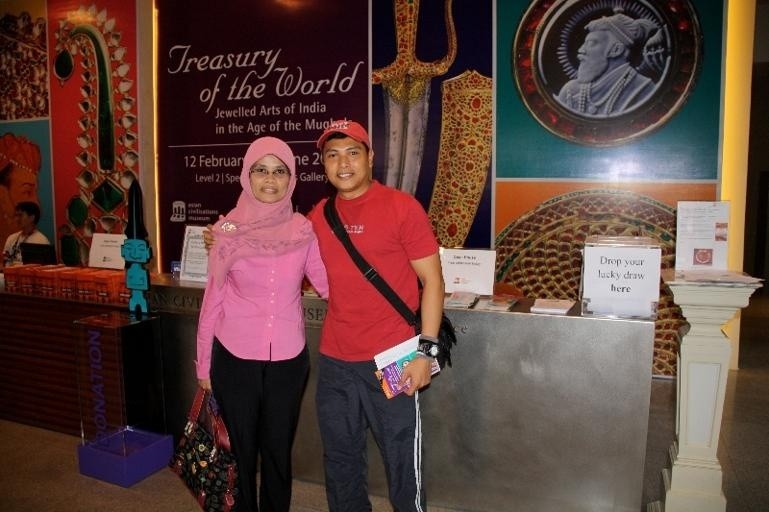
[1,258,658,511]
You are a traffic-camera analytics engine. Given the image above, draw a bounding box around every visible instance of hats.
[317,121,370,153]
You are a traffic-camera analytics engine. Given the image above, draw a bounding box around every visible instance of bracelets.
[416,335,440,362]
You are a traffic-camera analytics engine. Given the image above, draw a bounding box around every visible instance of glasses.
[249,167,290,178]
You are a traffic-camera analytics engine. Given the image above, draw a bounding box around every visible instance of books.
[446,294,576,315]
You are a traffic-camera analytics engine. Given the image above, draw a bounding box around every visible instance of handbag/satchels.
[167,386,238,512]
[416,306,456,369]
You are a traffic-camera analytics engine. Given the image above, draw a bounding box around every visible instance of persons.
[195,137,329,511]
[203,118,446,510]
[555,12,661,117]
[1,202,51,265]
[0,132,42,258]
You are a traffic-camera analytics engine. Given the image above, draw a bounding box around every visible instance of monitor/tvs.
[20,242,57,265]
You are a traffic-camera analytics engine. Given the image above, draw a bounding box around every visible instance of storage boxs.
[577,233,664,323]
